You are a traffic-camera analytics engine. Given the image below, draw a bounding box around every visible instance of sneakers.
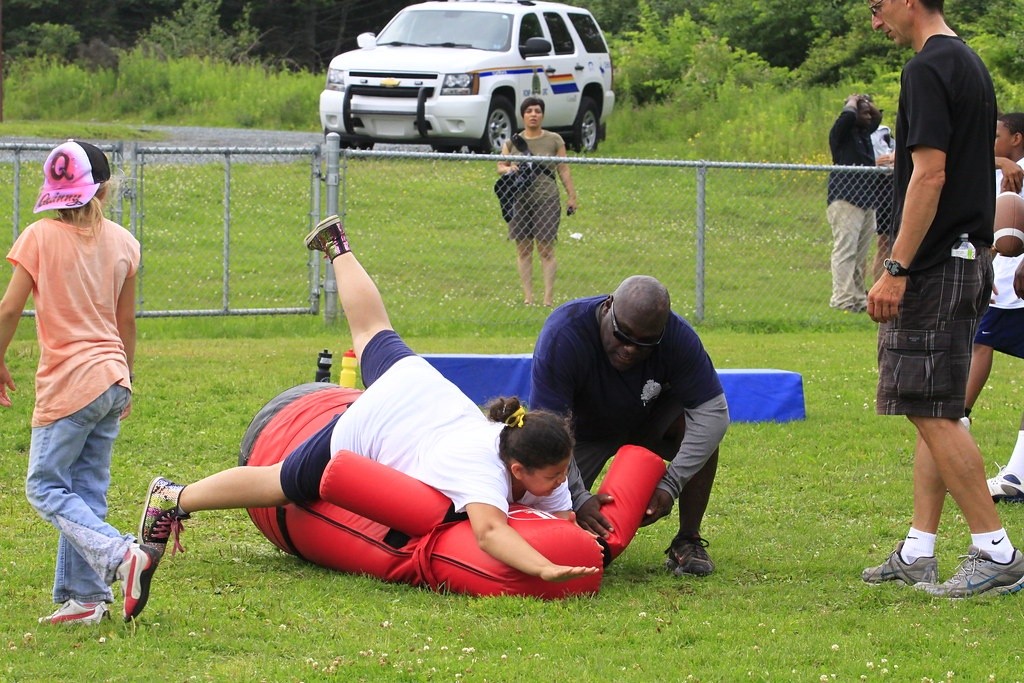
[982,462,1024,505]
[39,598,111,629]
[115,542,160,622]
[862,540,937,585]
[305,215,351,261]
[912,543,1024,602]
[665,531,715,580]
[959,408,973,431]
[139,476,191,557]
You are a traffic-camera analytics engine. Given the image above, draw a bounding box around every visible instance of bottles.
[314,349,332,383]
[339,349,359,389]
[950,233,976,260]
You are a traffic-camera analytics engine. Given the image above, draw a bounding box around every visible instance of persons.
[958,111,1024,431]
[861,0,1024,599]
[985,259,1024,502]
[872,125,895,284]
[497,97,577,306]
[0,139,159,624]
[137,213,604,579]
[828,93,883,312]
[530,273,730,578]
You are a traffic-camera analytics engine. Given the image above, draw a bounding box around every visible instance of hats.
[33,139,110,214]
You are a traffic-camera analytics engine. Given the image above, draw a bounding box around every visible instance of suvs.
[319,0,616,155]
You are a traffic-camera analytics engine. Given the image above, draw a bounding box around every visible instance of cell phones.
[567,208,573,215]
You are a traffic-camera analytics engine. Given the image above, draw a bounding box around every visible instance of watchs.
[884,258,910,276]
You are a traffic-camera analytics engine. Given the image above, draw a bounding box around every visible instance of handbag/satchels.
[494,171,516,221]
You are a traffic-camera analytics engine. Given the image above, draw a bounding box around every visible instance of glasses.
[609,302,665,349]
[868,0,883,16]
